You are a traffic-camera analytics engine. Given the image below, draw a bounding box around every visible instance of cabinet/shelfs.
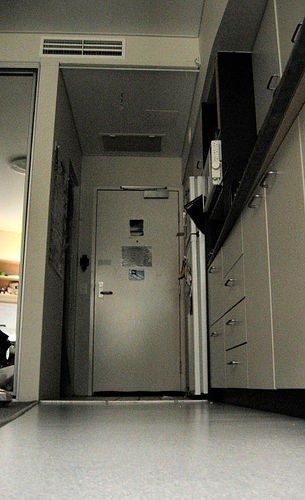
[221,208,251,409]
[251,0,305,135]
[0,258,19,304]
[201,52,257,234]
[241,115,305,420]
[207,245,224,405]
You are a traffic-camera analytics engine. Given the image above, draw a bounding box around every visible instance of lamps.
[8,157,27,175]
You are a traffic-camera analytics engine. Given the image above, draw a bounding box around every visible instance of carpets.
[0,401,38,427]
